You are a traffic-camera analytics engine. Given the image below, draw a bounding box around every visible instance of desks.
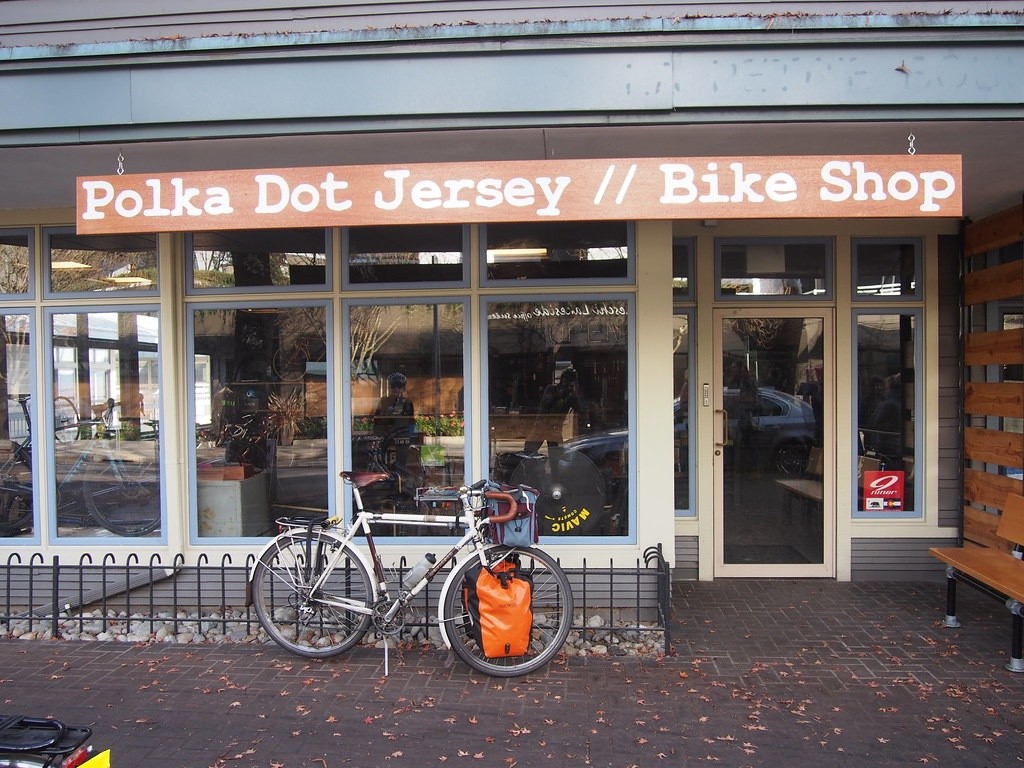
[416,487,464,536]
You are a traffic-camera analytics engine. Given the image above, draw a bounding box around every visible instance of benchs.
[928,493,1024,673]
[774,448,881,537]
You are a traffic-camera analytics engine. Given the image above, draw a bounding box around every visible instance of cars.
[562,384,816,506]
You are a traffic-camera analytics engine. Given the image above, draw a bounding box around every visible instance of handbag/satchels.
[461,555,533,657]
[484,480,540,548]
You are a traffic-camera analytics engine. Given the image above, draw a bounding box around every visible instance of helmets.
[388,373,407,385]
[561,368,577,380]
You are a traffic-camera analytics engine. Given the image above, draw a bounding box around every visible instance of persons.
[680,369,689,432]
[371,375,416,437]
[528,365,594,475]
[735,375,763,468]
[860,374,903,459]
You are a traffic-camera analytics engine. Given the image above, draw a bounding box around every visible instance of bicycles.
[248,471,574,676]
[53,395,80,444]
[147,411,299,478]
[0,391,162,537]
[0,714,111,768]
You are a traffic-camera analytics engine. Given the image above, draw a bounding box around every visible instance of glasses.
[391,384,403,389]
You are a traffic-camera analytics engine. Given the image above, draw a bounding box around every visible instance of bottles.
[401,553,436,590]
